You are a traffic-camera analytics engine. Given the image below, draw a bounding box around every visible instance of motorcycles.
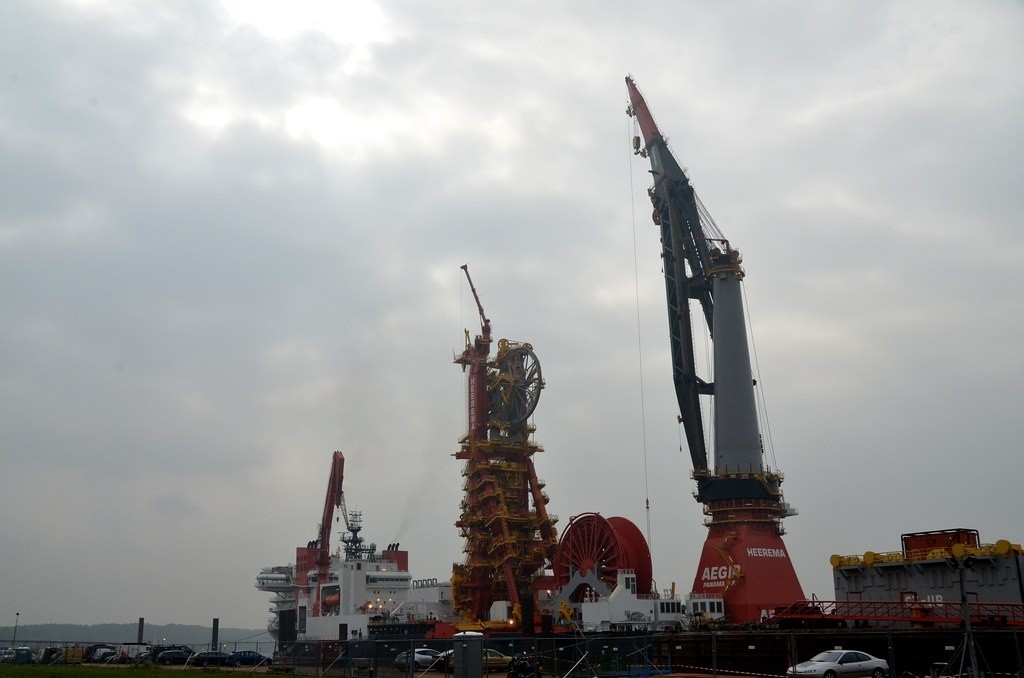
[506,643,546,678]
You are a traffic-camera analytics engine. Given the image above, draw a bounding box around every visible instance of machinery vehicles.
[255,450,513,665]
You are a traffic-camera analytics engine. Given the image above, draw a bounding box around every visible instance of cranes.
[621,73,819,631]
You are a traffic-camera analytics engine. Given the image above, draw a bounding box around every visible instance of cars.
[786,649,890,678]
[187,650,229,667]
[156,650,192,666]
[395,647,442,674]
[432,648,454,674]
[225,650,273,667]
[447,648,514,673]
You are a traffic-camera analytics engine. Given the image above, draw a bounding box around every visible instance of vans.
[0,642,197,666]
